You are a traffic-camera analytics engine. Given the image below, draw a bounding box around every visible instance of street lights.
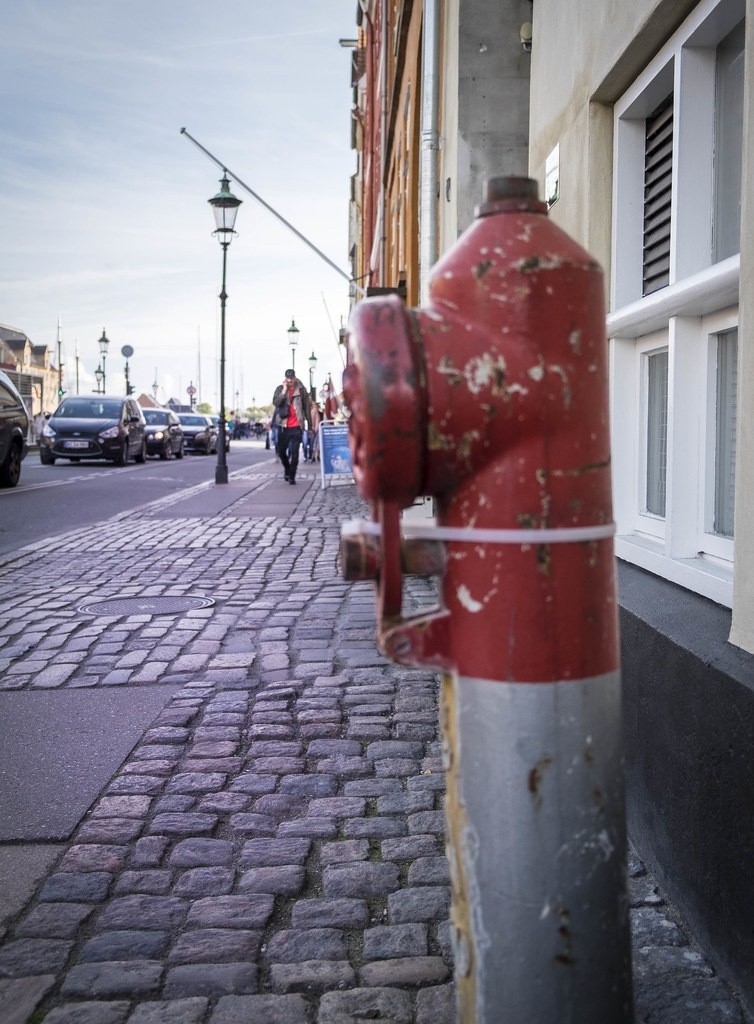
[307,351,321,405]
[206,166,243,486]
[97,327,111,395]
[287,318,301,373]
[95,365,103,395]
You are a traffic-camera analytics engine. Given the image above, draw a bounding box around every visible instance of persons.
[302,399,323,463]
[228,419,266,440]
[273,369,313,485]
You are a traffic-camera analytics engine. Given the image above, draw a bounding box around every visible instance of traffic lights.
[58,386,64,396]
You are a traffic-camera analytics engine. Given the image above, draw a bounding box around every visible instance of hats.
[285,370,294,378]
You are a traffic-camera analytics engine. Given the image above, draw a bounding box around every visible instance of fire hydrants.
[336,170,637,1023]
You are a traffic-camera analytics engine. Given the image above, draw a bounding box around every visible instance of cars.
[38,392,149,467]
[201,414,234,454]
[160,412,217,457]
[139,407,185,461]
[0,370,32,490]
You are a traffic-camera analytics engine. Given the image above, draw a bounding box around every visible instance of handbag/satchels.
[279,404,290,418]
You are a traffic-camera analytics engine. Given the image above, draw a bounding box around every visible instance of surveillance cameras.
[520,21,533,53]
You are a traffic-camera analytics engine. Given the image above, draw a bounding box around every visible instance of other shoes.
[289,479,295,484]
[284,468,290,480]
